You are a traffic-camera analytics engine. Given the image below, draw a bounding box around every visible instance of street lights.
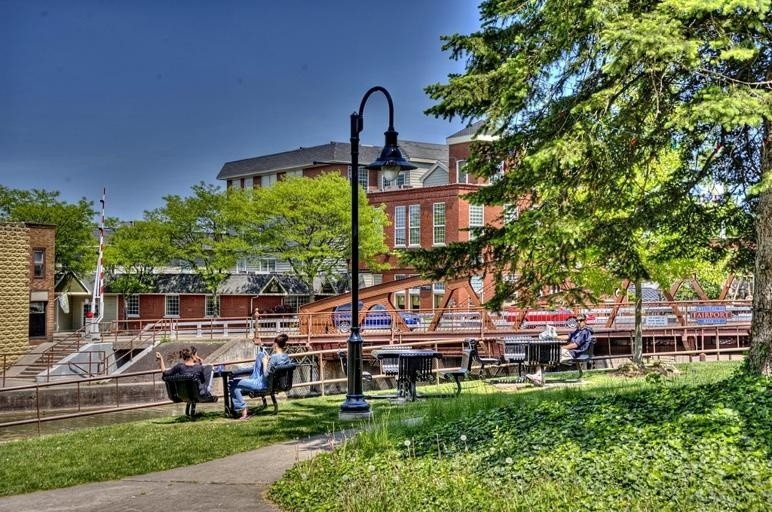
[341,84,418,410]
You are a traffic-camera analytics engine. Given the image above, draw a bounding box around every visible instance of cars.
[249,308,274,327]
[504,297,598,329]
[273,304,293,326]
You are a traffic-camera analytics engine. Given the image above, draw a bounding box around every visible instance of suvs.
[333,301,418,333]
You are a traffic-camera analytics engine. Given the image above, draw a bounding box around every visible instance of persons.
[525,313,595,387]
[230,334,293,419]
[155,345,218,402]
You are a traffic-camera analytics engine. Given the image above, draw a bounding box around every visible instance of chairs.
[378,342,412,378]
[438,344,476,398]
[238,360,296,417]
[395,351,439,401]
[470,336,594,386]
[163,371,217,420]
[338,347,375,383]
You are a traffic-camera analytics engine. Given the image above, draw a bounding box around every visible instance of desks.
[211,367,259,414]
[371,345,422,379]
